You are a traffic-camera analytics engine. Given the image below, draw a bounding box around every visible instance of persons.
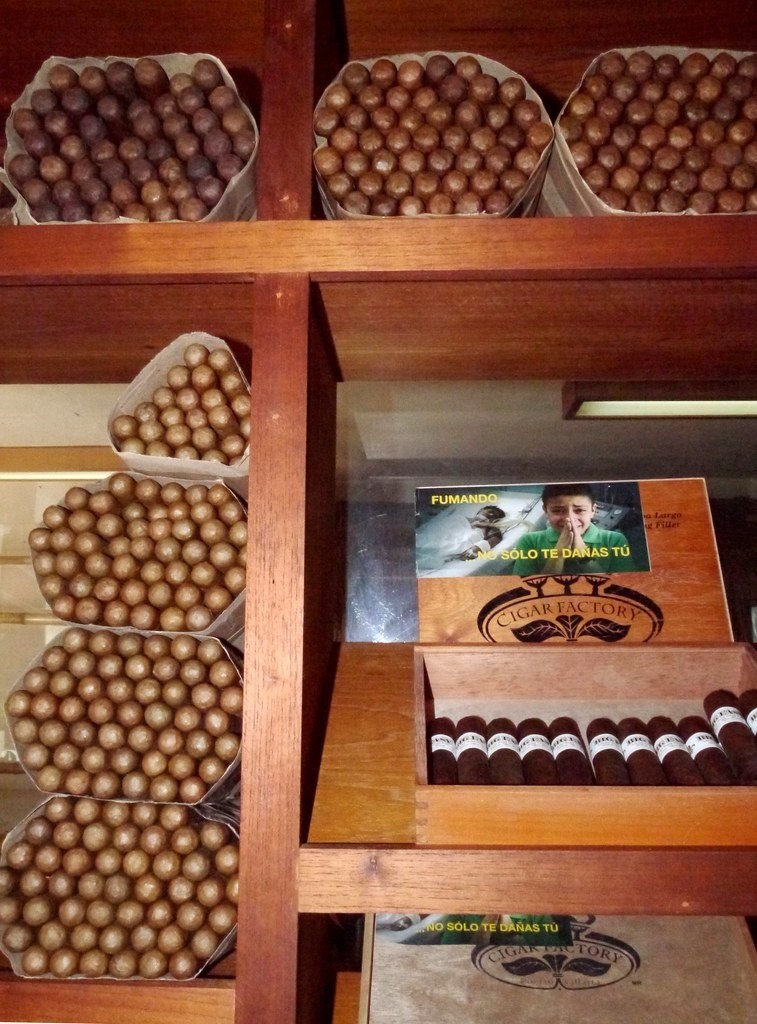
[462,506,505,561]
[512,484,638,576]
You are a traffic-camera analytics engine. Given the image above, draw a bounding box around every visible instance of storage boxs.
[560,380,757,420]
[412,478,757,847]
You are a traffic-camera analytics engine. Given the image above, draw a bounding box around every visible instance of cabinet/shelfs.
[0,0,757,1024]
